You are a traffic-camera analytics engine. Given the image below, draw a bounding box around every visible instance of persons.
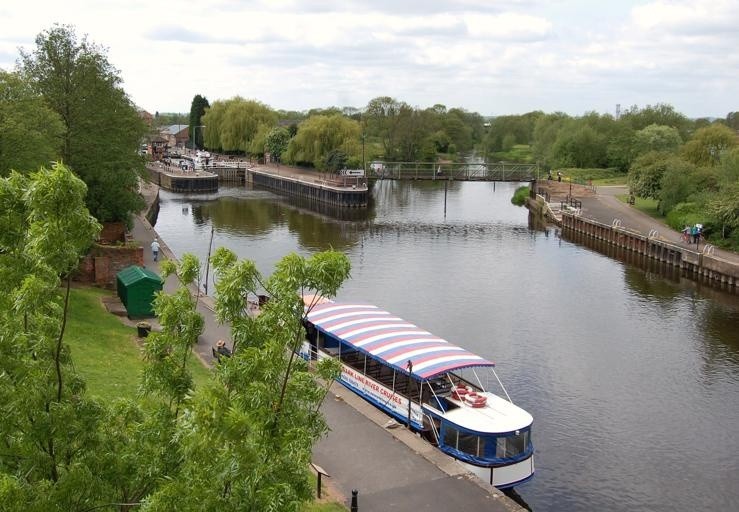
[691,224,697,243]
[557,170,563,182]
[215,340,232,358]
[150,238,160,261]
[547,169,553,180]
[681,225,691,244]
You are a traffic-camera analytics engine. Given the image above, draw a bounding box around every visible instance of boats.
[257,291,537,491]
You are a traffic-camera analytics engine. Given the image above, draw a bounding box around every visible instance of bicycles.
[680,230,690,244]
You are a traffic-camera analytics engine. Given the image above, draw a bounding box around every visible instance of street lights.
[192,125,207,156]
[500,161,505,181]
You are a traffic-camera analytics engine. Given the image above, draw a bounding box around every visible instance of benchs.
[211,346,232,364]
[341,350,460,402]
[567,194,581,209]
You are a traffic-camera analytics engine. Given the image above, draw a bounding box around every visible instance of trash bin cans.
[137,322,151,337]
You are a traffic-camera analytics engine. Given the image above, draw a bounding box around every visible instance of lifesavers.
[451,384,487,408]
